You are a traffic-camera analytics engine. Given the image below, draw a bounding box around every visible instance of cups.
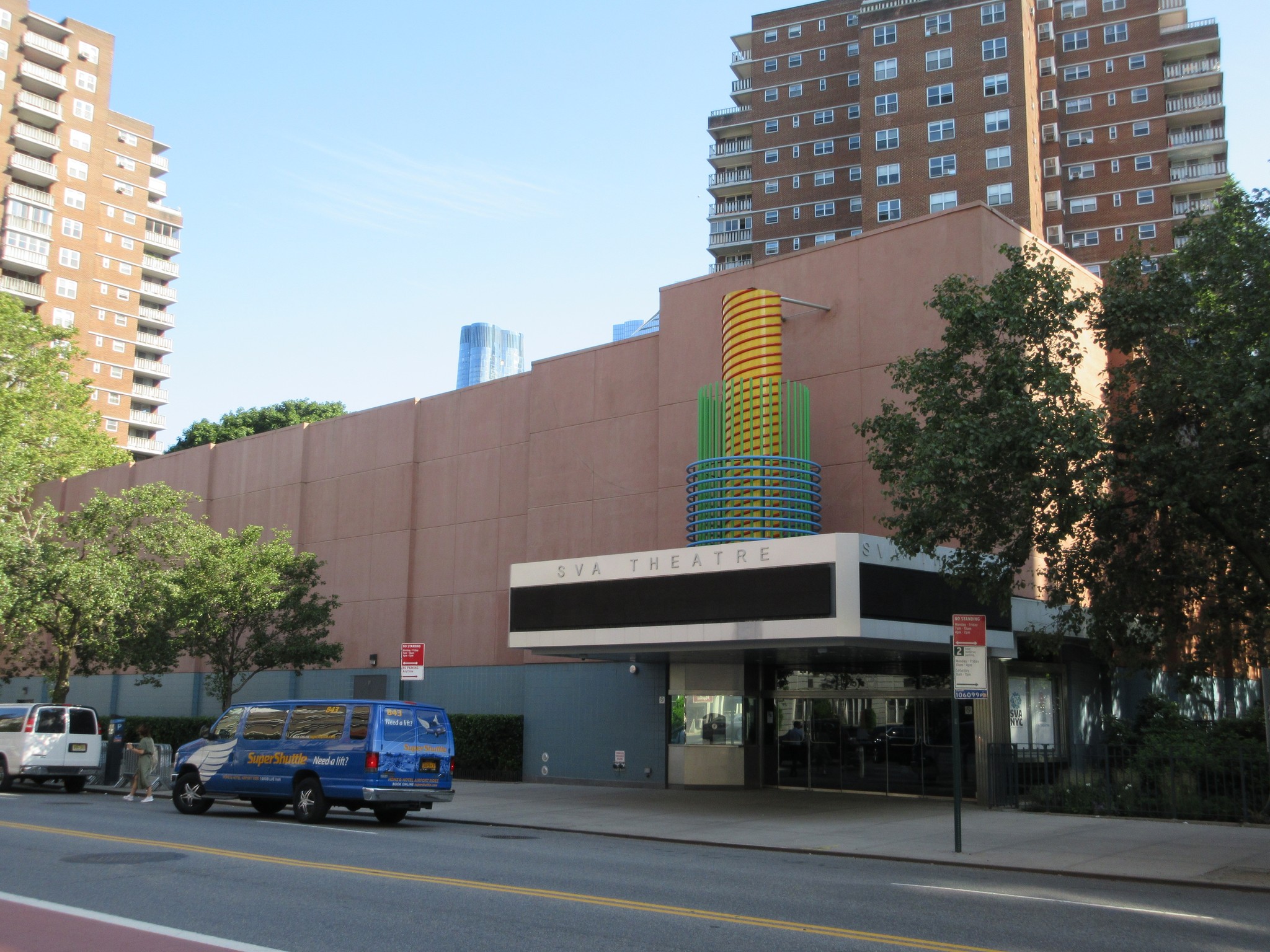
[126,742,132,750]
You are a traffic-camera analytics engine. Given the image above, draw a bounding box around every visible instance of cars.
[847,724,926,766]
[700,713,742,741]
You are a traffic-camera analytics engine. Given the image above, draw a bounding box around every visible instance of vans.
[0,703,101,794]
[170,699,455,825]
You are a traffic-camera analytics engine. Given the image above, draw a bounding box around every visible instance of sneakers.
[141,795,154,802]
[123,794,134,802]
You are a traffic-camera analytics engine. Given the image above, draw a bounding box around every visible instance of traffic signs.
[401,643,425,680]
[953,614,988,699]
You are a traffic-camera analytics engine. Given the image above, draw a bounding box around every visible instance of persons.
[123,726,155,802]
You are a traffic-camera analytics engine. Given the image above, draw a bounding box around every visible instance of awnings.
[509,532,1013,665]
